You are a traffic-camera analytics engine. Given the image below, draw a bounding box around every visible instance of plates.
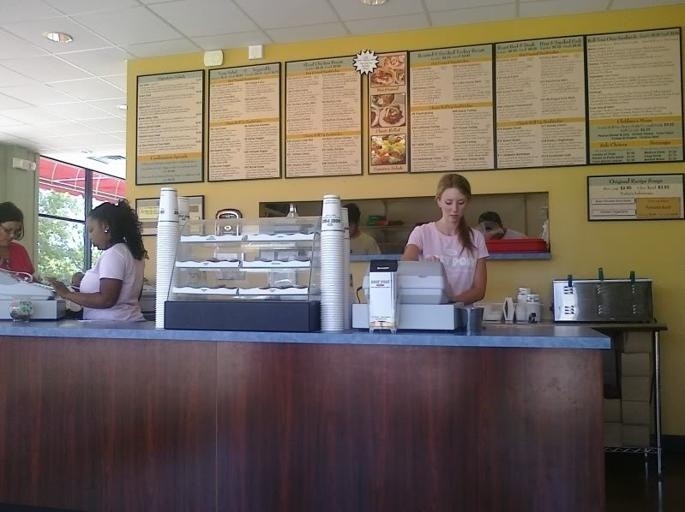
[370,94,406,127]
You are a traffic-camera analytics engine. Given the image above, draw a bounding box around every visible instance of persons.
[400,173,492,305]
[343,203,381,255]
[51,198,149,321]
[56,272,85,319]
[0,202,36,281]
[479,212,525,239]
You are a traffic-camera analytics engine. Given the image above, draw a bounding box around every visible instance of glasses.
[0,223,22,237]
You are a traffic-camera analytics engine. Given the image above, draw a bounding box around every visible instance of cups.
[320,195,345,332]
[154,187,194,330]
[339,206,352,329]
[515,287,542,323]
